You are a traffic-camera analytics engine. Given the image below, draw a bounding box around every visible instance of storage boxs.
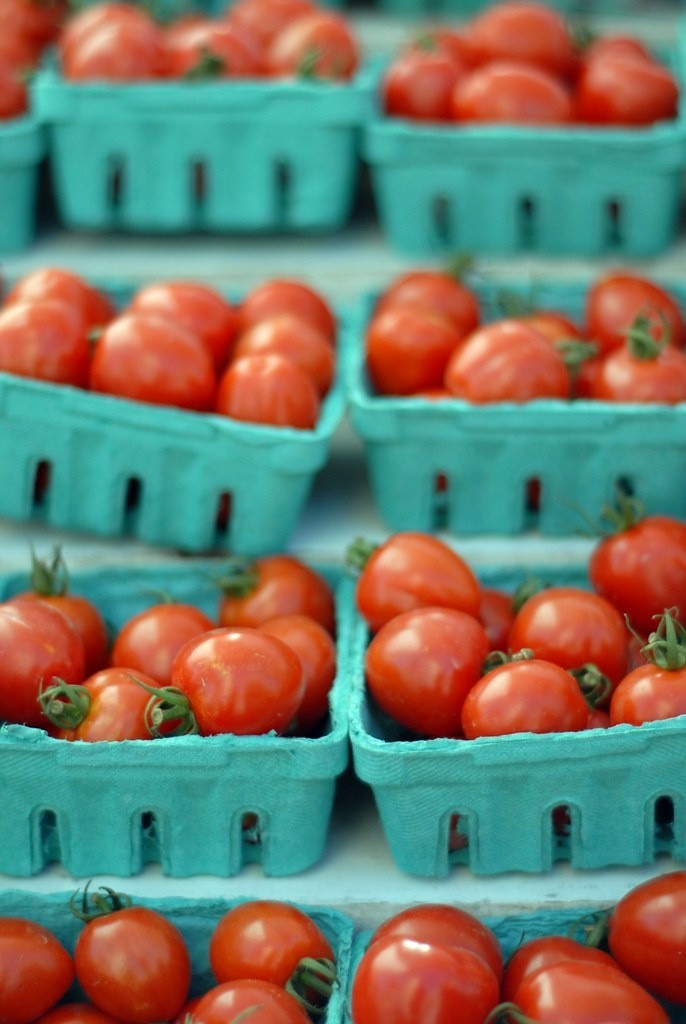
[360,44,686,262]
[0,565,360,877]
[344,906,611,1024]
[0,276,351,560]
[347,559,686,878]
[28,16,373,237]
[347,278,686,538]
[0,887,354,1024]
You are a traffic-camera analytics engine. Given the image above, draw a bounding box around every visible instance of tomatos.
[0,266,338,520]
[0,867,686,1024]
[357,517,686,741]
[0,552,337,743]
[366,268,686,512]
[0,0,679,132]
